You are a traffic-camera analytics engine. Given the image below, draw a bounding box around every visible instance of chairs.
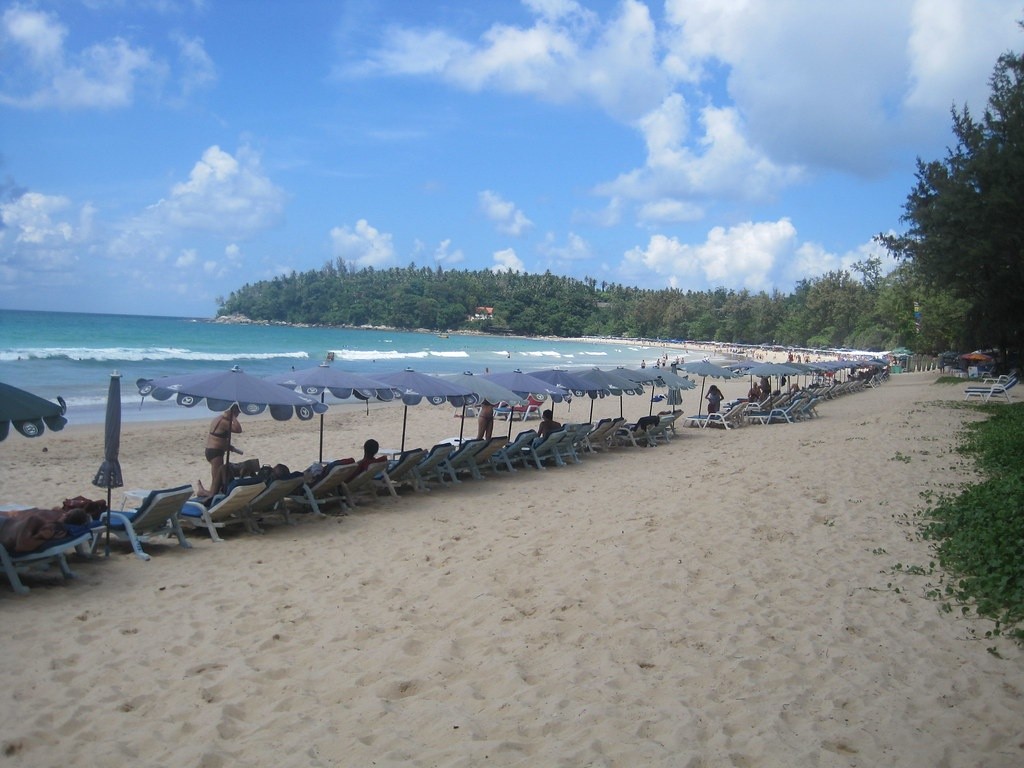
[0,457,358,596]
[964,366,1018,403]
[438,394,684,479]
[349,460,401,500]
[369,447,428,497]
[684,370,887,430]
[417,442,459,488]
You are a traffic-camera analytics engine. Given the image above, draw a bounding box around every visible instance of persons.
[197,340,906,504]
[0,495,106,552]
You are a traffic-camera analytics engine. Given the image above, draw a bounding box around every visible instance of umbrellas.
[92,370,124,556]
[0,383,68,442]
[136,358,886,493]
